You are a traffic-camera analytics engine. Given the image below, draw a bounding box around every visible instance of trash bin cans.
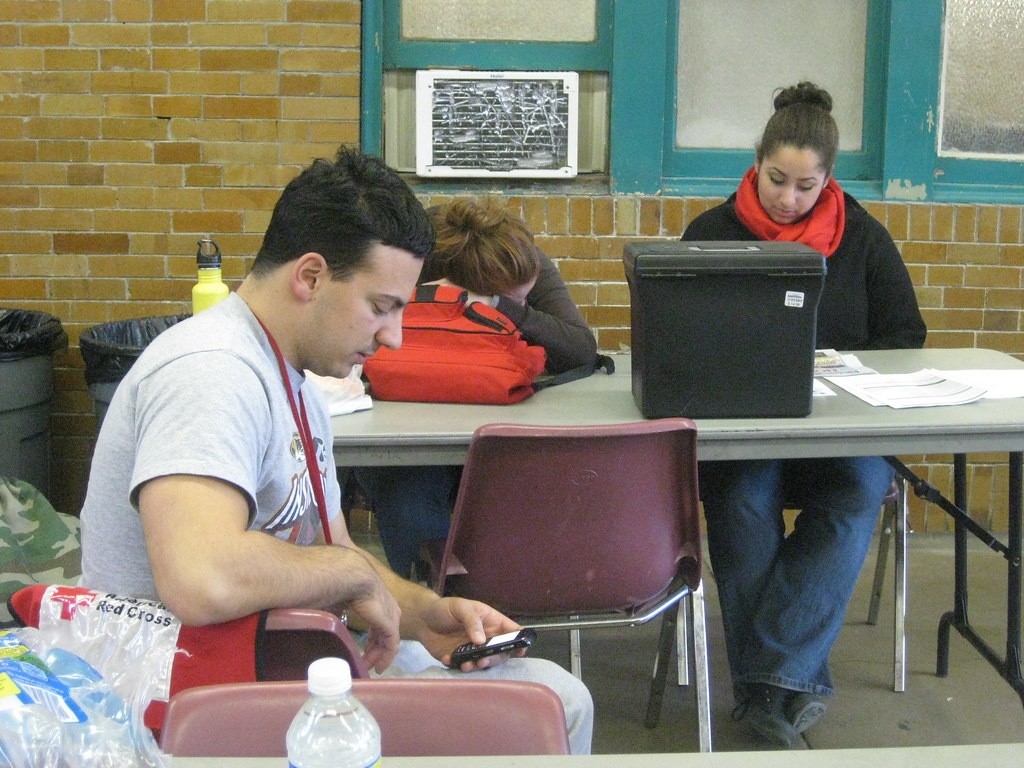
[79,313,194,435]
[0,307,70,503]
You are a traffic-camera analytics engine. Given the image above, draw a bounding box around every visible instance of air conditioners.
[414,70,577,179]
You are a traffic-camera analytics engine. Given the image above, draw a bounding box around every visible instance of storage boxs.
[621,240,827,418]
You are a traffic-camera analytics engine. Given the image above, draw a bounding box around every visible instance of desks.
[328,348,1024,700]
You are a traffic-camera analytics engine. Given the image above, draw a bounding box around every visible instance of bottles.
[192,241,229,317]
[286,658,381,768]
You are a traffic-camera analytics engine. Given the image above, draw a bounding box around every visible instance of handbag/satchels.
[363,293,613,404]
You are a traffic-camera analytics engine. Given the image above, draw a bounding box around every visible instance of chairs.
[6,584,571,756]
[676,470,908,692]
[436,416,712,754]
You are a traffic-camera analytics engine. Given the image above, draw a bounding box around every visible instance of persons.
[680,83,927,748]
[79,144,596,755]
[352,198,598,579]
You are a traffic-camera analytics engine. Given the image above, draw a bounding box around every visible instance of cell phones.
[450,627,537,664]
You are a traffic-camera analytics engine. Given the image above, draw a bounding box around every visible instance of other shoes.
[734,674,826,735]
[731,685,797,749]
